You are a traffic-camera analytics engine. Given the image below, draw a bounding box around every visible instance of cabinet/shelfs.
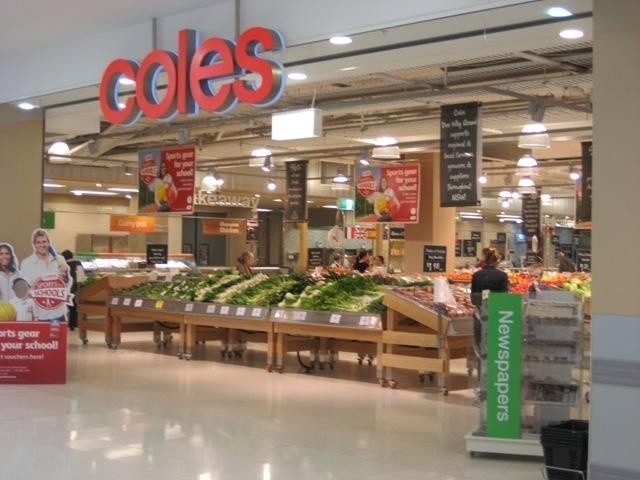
[74,274,475,398]
[462,289,585,459]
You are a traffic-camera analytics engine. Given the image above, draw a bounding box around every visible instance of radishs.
[195,271,270,303]
[278,279,333,307]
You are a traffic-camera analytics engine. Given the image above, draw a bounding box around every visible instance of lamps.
[479,94,580,208]
[42,85,401,193]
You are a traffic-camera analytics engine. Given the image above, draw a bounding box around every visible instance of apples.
[388,201,397,212]
[167,189,174,201]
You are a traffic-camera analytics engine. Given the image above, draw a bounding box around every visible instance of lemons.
[377,198,388,212]
[157,184,166,200]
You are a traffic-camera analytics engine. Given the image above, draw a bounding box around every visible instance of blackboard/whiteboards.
[423,245,446,273]
[455,231,480,257]
[308,248,322,269]
[146,244,168,268]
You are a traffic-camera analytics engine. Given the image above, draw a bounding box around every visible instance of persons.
[236,251,255,276]
[478,248,491,269]
[469,249,508,343]
[373,176,400,221]
[154,161,178,212]
[60,249,86,330]
[20,227,69,321]
[8,277,38,322]
[330,249,387,275]
[557,252,575,272]
[0,243,30,302]
